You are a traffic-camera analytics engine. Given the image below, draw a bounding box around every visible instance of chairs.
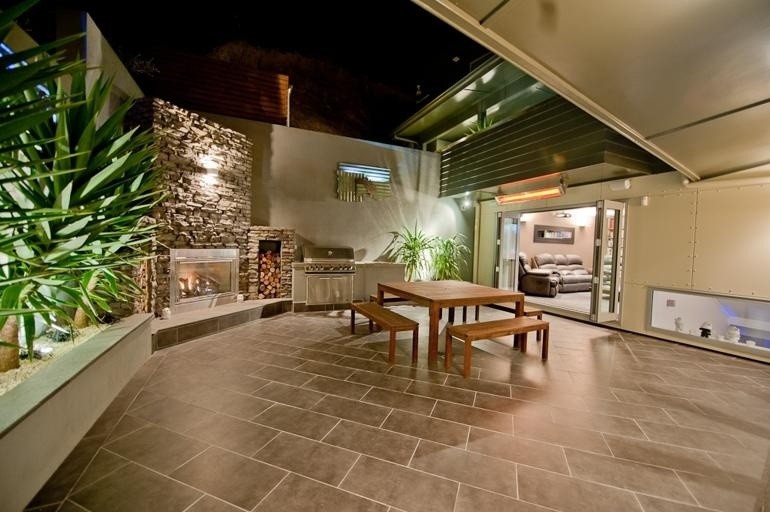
[518,253,560,298]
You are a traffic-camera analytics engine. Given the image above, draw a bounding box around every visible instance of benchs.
[350,301,419,366]
[462,302,542,341]
[370,293,442,320]
[445,317,549,378]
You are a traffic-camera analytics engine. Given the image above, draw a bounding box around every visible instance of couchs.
[531,254,592,293]
